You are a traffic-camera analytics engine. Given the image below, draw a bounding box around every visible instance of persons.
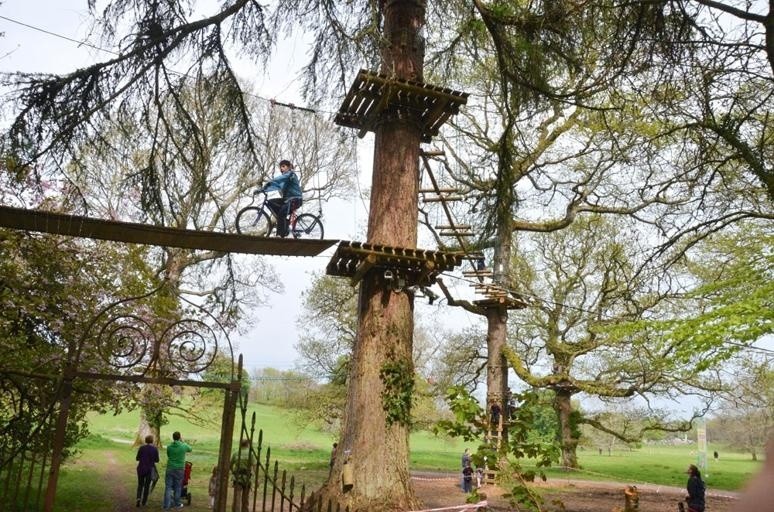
[254,160,304,239]
[135,434,159,507]
[489,401,504,426]
[506,390,519,421]
[463,462,474,494]
[207,465,217,509]
[330,442,338,465]
[684,465,706,512]
[475,467,484,488]
[460,447,474,493]
[230,437,261,511]
[624,485,639,512]
[162,431,193,509]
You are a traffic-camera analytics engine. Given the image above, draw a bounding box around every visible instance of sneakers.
[136,498,140,507]
[177,504,183,509]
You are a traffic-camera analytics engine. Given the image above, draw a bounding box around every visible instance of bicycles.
[235,189,324,240]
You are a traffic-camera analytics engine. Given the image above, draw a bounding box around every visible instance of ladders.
[485,405,504,486]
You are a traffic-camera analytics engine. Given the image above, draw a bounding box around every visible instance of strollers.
[171,461,192,506]
